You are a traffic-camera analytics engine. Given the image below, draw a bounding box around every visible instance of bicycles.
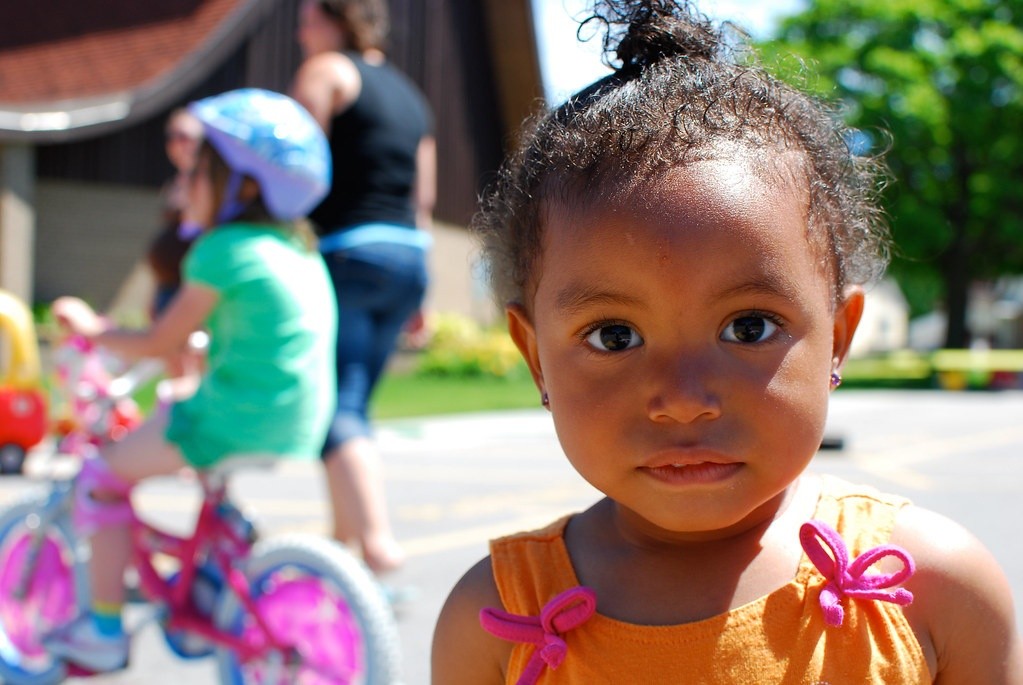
[0,333,407,684]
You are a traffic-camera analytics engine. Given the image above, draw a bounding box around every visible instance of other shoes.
[41,614,128,672]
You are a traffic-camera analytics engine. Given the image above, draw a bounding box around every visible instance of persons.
[431,0,1023,685]
[143,90,207,388]
[293,0,437,610]
[41,90,339,671]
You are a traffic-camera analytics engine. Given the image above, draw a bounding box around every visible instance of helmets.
[188,87,333,222]
